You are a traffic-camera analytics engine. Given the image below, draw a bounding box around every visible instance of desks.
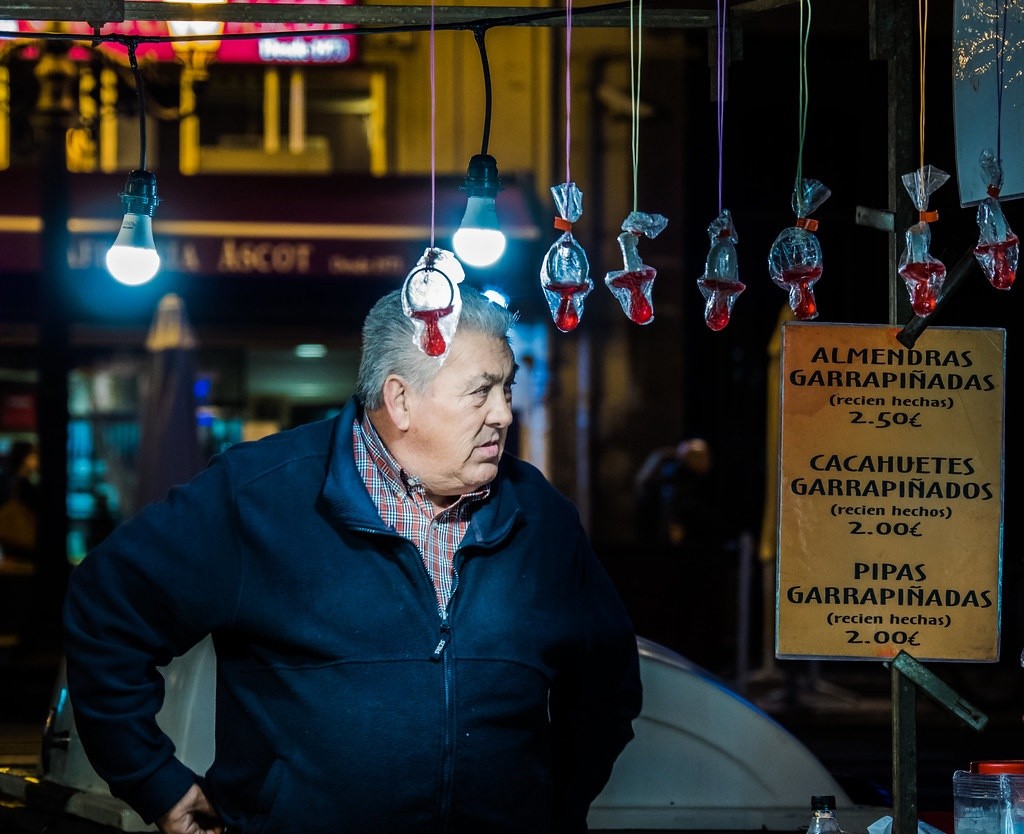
[87,59,399,171]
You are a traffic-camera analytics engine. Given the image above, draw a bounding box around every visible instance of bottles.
[805,794,842,834]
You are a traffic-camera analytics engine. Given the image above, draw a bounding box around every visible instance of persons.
[63,286,644,834]
